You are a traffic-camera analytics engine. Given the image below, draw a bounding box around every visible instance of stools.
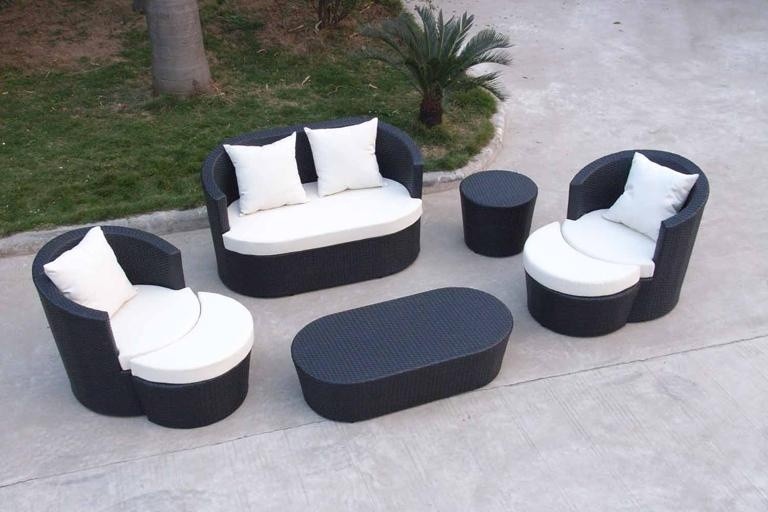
[130,287,255,429]
[459,169,537,257]
[521,220,640,338]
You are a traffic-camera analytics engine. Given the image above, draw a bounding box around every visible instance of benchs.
[202,117,425,298]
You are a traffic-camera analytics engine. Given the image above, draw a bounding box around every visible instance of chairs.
[32,226,185,428]
[569,150,710,338]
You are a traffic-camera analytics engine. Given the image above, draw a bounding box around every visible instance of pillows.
[42,226,136,318]
[220,117,386,216]
[600,150,698,243]
